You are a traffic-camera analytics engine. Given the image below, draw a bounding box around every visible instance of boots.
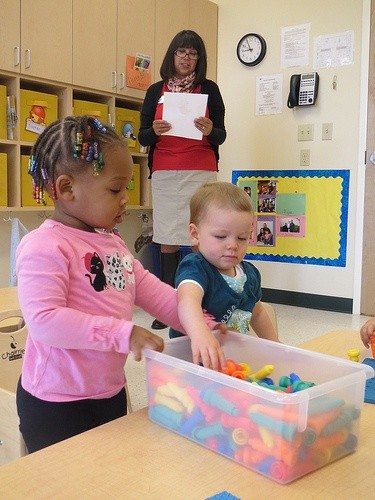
[151,249,181,329]
[169,328,185,338]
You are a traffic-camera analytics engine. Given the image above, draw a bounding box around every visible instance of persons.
[138,30,227,329]
[16,115,245,454]
[260,182,275,195]
[169,181,284,372]
[259,197,275,212]
[360,317,375,348]
[283,220,295,232]
[257,223,273,246]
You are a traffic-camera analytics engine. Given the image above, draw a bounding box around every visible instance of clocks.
[237,33,267,67]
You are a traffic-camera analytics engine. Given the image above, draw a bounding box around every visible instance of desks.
[0,329,375,500]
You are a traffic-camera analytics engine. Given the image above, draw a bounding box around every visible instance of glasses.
[173,50,200,60]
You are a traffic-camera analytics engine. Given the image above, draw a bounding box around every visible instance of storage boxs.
[143,331,374,485]
[20,155,55,208]
[20,89,59,142]
[128,164,140,205]
[0,85,8,140]
[74,99,109,125]
[0,153,8,208]
[114,106,147,154]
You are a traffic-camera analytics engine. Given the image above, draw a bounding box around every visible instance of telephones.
[285,72,318,107]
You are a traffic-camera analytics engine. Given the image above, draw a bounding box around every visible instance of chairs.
[0,310,132,461]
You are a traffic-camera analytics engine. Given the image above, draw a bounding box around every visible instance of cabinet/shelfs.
[0,0,73,86]
[156,0,218,83]
[0,72,154,212]
[73,0,156,100]
